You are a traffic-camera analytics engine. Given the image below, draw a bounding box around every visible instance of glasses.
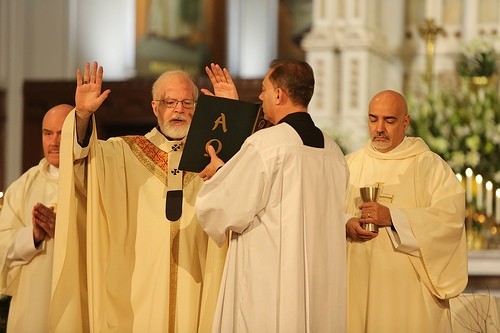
[155,97,197,109]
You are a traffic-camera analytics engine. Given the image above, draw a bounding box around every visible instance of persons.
[48,62,238,333]
[0,105,75,333]
[196,59,351,333]
[345,90,468,333]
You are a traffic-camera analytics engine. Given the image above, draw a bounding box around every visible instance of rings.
[83,81,89,84]
[367,212,371,217]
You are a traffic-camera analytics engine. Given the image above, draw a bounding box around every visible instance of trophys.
[360,187,380,232]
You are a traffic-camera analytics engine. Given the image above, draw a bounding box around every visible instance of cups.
[360,187,380,233]
